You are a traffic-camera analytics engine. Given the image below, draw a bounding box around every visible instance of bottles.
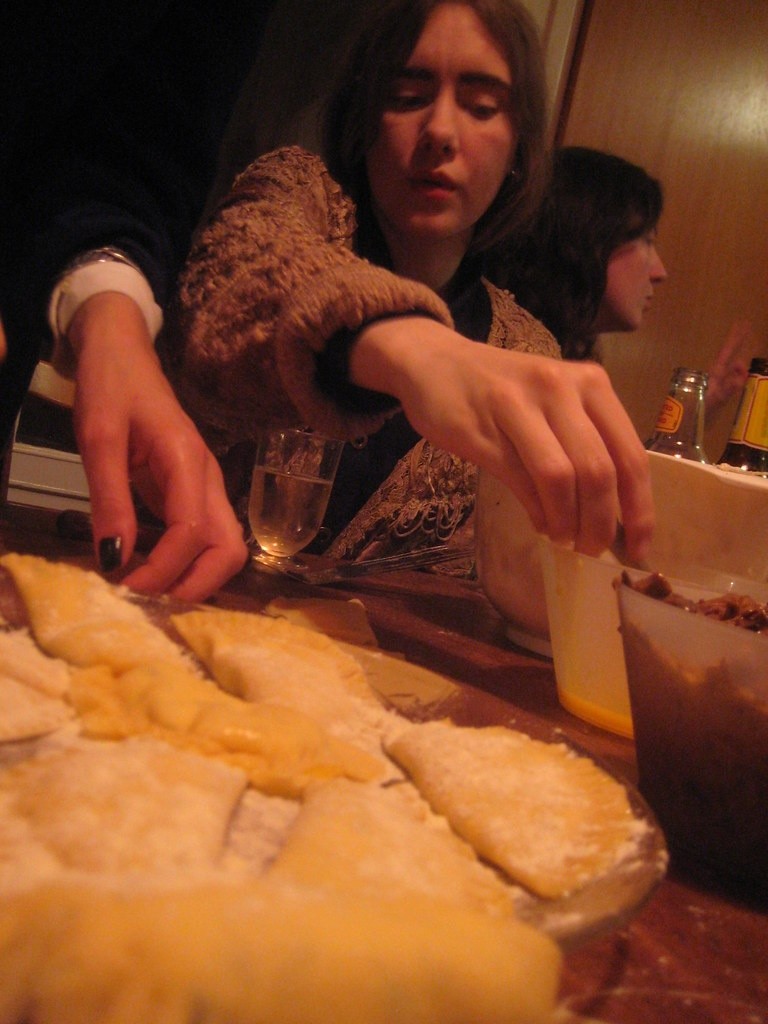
[717,357,768,477]
[643,367,711,465]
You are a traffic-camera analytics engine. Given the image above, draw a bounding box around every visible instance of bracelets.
[53,246,148,281]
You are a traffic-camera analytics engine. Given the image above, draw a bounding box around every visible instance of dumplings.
[0,550,643,1024]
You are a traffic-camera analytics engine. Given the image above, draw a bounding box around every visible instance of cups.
[248,427,343,573]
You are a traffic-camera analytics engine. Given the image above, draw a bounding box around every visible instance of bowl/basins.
[475,427,768,661]
[538,527,768,916]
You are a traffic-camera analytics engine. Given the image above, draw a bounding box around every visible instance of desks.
[0,508,768,1024]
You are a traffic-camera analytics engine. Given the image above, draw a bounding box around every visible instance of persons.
[174,1,664,582]
[483,144,752,460]
[0,1,250,619]
